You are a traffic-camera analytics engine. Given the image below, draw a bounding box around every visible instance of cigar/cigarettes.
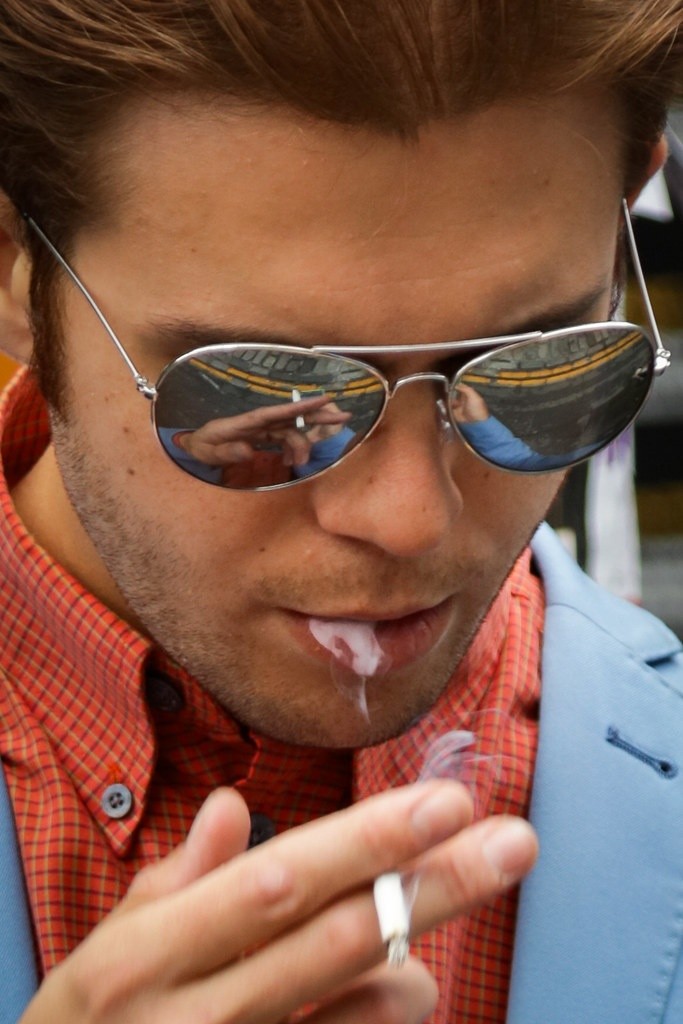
[292,389,305,429]
[373,872,410,968]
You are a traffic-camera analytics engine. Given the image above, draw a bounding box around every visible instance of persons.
[158,391,354,485]
[0,0,683,1024]
[450,383,613,472]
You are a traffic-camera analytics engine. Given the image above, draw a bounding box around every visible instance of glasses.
[22,194,667,491]
